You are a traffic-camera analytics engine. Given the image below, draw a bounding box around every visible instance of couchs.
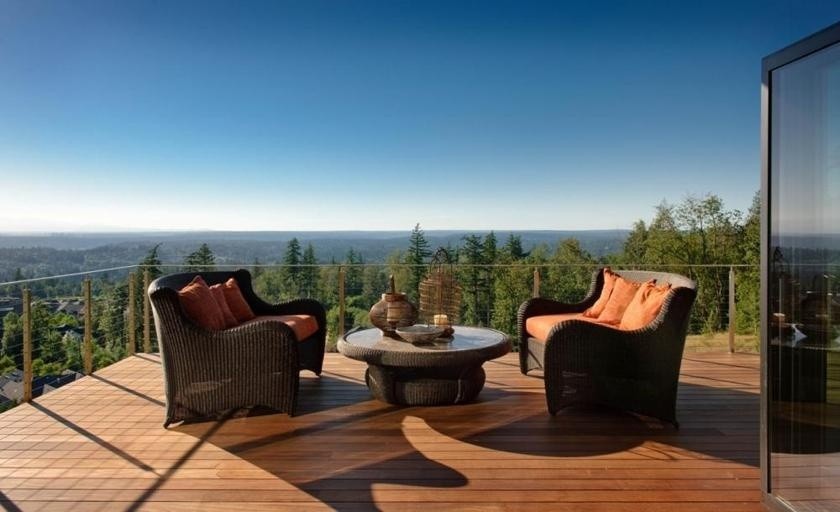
[517,268,698,431]
[148,268,327,428]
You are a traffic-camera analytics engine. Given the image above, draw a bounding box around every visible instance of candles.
[433,314,448,325]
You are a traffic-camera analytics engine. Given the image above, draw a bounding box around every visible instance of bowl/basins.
[395,326,443,345]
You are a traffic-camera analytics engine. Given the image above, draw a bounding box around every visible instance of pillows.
[173,274,257,331]
[583,267,672,331]
